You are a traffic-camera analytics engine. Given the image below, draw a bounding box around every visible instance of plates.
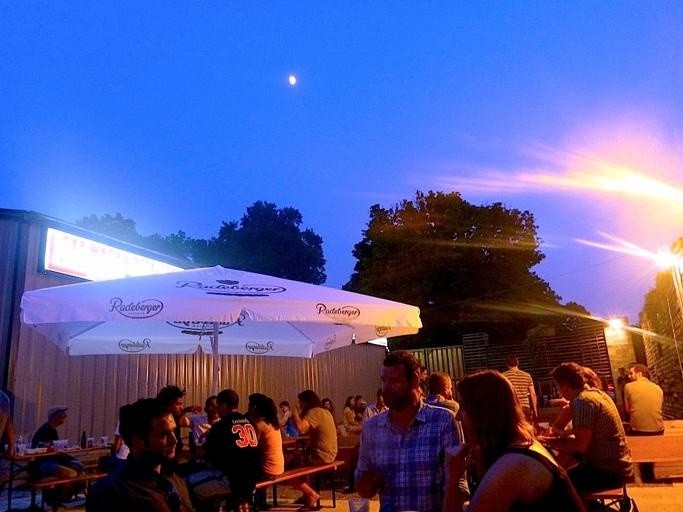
[33,448,47,454]
[24,449,34,455]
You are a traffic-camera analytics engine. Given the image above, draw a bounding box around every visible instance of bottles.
[80,431,87,449]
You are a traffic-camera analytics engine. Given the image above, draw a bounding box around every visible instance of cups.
[87,438,95,447]
[100,436,108,446]
[52,440,63,452]
[61,439,69,450]
[538,422,549,437]
[15,439,25,457]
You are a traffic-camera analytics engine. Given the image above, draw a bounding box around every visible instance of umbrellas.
[18,264,423,399]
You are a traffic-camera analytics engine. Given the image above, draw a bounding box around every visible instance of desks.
[280,437,312,450]
[7,447,114,511]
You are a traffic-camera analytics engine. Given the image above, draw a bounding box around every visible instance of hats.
[48,407,66,417]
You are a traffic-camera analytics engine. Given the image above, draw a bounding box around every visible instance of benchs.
[8,463,104,481]
[32,471,107,512]
[590,488,624,511]
[624,436,681,463]
[254,460,345,508]
